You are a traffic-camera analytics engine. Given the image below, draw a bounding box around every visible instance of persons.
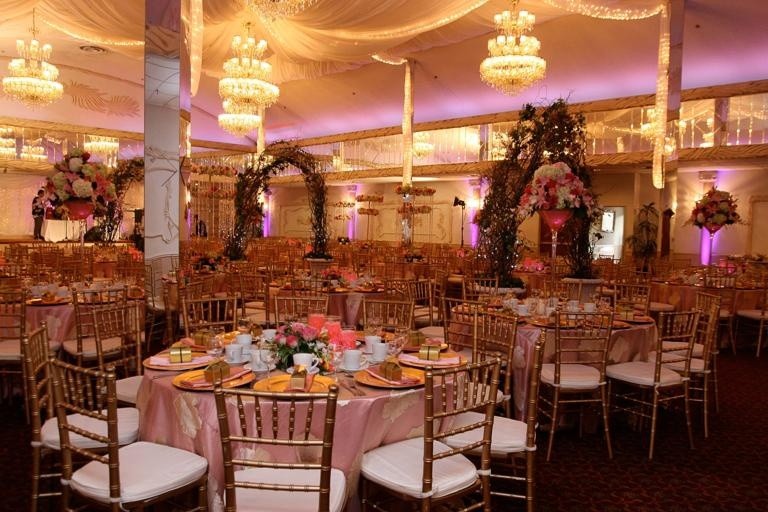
[193,214,207,236]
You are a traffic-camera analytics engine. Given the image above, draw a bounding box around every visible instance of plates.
[171,365,257,392]
[398,352,468,369]
[171,303,408,401]
[401,342,448,351]
[143,351,219,371]
[354,364,431,388]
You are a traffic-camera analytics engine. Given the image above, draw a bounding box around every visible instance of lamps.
[216,20,281,143]
[1,8,66,109]
[477,0,549,97]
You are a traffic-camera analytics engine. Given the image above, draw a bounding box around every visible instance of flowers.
[31,146,117,212]
[509,153,595,217]
[680,183,753,234]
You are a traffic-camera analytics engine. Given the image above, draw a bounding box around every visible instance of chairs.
[16,320,151,511]
[1,228,768,460]
[50,354,208,510]
[210,378,348,510]
[439,326,548,512]
[359,355,504,511]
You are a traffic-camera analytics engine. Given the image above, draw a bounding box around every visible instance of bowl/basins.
[31,280,122,302]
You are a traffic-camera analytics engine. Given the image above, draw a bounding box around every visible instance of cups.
[505,296,601,320]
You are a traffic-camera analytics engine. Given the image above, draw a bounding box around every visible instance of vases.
[61,199,94,219]
[536,208,574,227]
[704,224,724,233]
